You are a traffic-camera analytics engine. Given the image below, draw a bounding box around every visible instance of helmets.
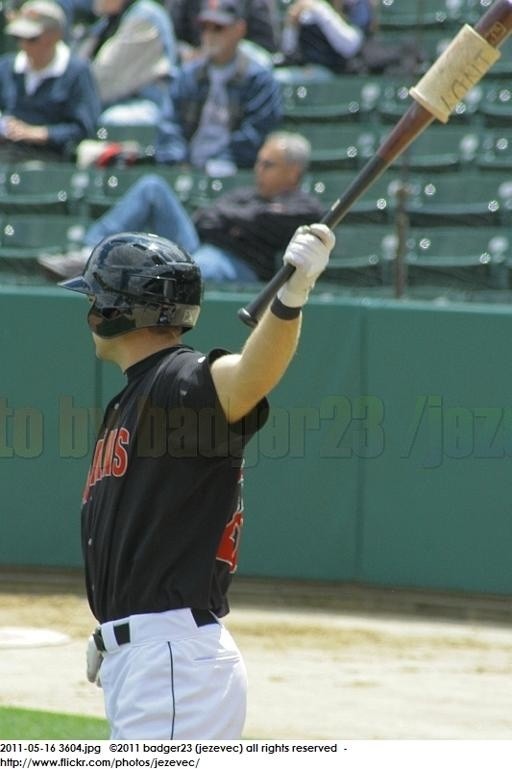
[55,230,202,335]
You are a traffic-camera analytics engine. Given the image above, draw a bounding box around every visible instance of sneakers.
[36,250,85,279]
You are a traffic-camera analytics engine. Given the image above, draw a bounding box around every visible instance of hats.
[3,1,68,40]
[192,1,242,30]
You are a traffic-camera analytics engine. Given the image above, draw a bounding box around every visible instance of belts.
[94,609,222,652]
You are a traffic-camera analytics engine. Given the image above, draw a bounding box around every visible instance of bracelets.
[268,295,302,322]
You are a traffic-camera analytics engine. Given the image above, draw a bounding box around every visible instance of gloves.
[269,222,336,320]
[85,632,103,686]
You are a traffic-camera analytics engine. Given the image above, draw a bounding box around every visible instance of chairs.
[2,3,512,301]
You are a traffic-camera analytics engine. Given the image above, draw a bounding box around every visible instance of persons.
[0,0,382,174]
[36,130,326,292]
[54,221,337,740]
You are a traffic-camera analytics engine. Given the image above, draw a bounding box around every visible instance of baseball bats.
[237,1,512,329]
